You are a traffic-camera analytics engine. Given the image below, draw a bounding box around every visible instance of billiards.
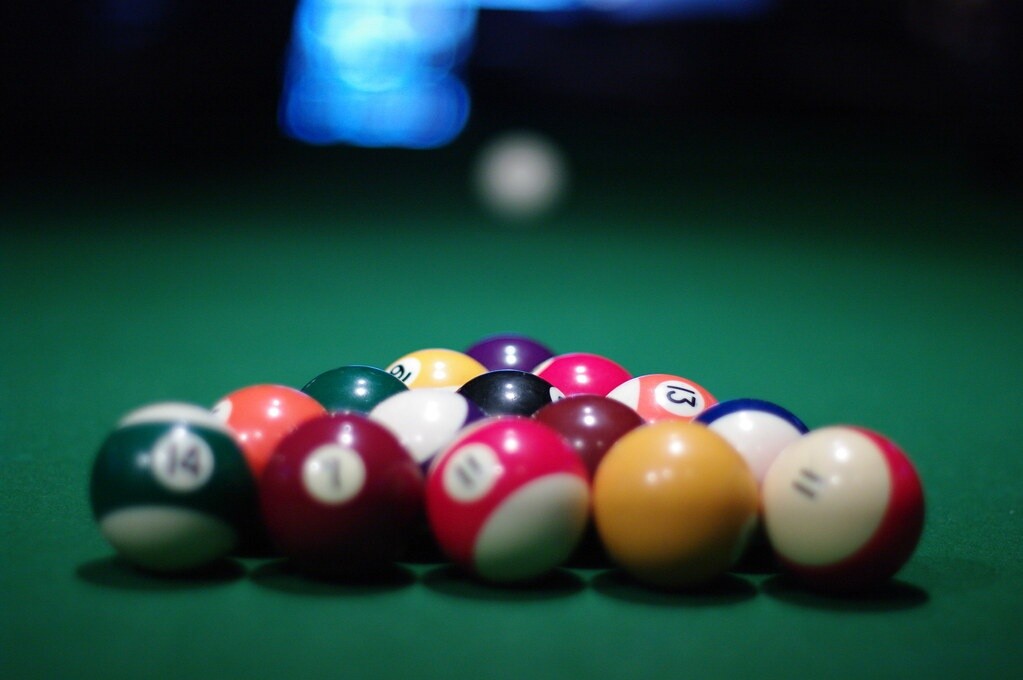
[82,334,930,595]
[478,136,567,224]
